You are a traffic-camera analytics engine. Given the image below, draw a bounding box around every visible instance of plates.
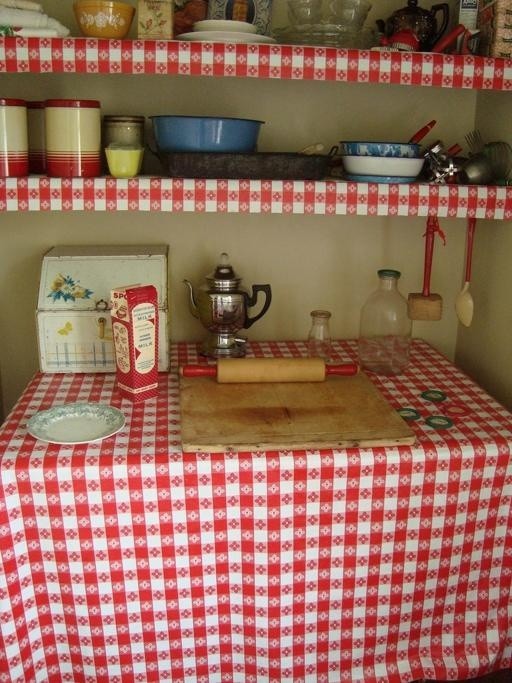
[173,30,278,47]
[273,22,376,49]
[191,18,259,33]
[25,402,128,445]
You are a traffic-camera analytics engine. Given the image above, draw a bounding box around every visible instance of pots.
[146,144,339,179]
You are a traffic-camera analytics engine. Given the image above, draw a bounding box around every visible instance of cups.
[104,142,145,179]
[460,152,494,183]
[101,112,145,146]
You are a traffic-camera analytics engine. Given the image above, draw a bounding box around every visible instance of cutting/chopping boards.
[176,368,419,453]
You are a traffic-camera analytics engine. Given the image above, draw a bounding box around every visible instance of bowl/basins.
[339,137,428,183]
[71,0,137,39]
[145,114,266,152]
[285,0,373,29]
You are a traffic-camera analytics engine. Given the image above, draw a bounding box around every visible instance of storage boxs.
[34,241,173,374]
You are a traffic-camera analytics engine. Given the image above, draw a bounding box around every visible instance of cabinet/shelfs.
[1,0,512,223]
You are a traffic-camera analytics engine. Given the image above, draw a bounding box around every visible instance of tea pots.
[181,251,275,358]
[375,0,450,49]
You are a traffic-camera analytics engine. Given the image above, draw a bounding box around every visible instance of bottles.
[308,309,334,359]
[356,267,415,374]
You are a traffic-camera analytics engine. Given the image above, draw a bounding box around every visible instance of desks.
[1,336,511,682]
